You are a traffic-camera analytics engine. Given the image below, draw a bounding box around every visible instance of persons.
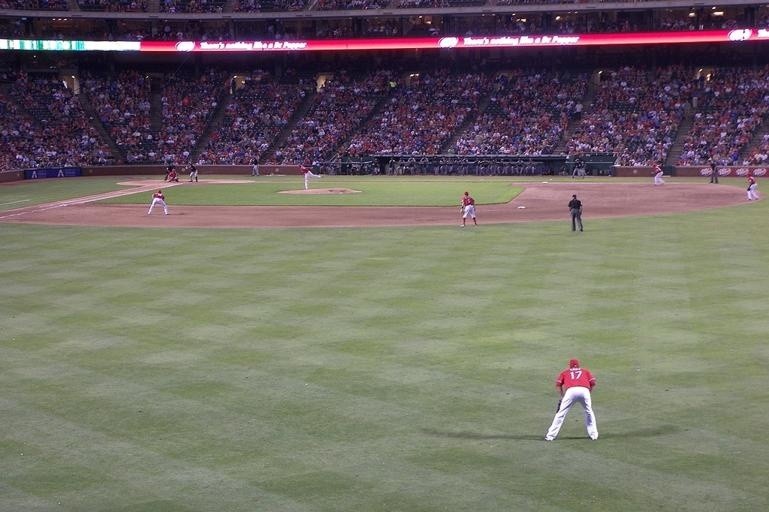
[746,172,760,200]
[0,0,769,186]
[568,194,585,231]
[544,358,599,443]
[460,192,478,226]
[148,189,168,215]
[299,164,324,191]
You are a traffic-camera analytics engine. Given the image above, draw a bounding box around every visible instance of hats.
[568,358,579,367]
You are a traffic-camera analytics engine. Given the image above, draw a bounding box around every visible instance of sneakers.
[545,436,599,441]
